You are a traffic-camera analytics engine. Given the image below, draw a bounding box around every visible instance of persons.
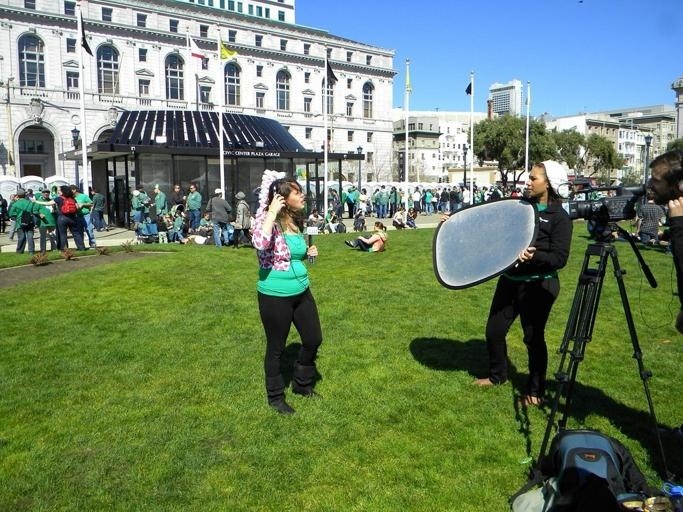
[130,182,262,250]
[308,185,523,233]
[248,177,322,414]
[345,222,388,254]
[441,160,573,408]
[0,185,109,256]
[636,193,664,246]
[588,209,636,241]
[577,182,614,198]
[649,149,683,336]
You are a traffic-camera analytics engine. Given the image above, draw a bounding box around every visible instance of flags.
[406,74,411,93]
[81,15,94,56]
[465,84,472,95]
[220,39,237,62]
[328,60,339,87]
[190,36,205,58]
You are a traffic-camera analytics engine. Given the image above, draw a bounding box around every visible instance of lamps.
[252,141,264,148]
[156,135,167,144]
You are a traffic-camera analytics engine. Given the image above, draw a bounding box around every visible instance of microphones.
[303,222,318,263]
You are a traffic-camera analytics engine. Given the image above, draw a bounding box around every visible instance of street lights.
[642,134,652,204]
[511,158,517,189]
[355,146,363,192]
[70,126,80,187]
[461,146,469,187]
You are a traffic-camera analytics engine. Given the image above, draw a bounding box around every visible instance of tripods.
[528,242,670,490]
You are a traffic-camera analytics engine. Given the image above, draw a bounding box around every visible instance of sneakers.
[265,392,294,415]
[293,384,322,401]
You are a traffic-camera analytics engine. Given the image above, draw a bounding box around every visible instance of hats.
[17,184,58,196]
[214,186,246,200]
[132,183,160,196]
[542,160,573,200]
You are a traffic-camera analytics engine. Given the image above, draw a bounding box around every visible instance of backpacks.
[551,429,623,512]
[61,195,77,214]
[20,212,36,231]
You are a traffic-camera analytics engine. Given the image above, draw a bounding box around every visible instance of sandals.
[343,239,355,249]
[472,374,507,388]
[517,393,546,409]
